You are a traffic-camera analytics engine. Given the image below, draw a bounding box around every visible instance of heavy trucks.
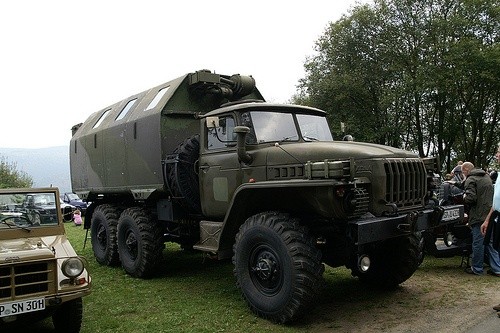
[70,69,474,324]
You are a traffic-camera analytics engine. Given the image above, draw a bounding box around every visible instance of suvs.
[0,187,92,333]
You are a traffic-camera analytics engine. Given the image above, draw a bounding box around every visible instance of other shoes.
[464,267,477,275]
[487,269,500,277]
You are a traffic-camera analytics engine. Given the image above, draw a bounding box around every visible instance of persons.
[460,161,500,277]
[444,160,468,190]
[479,142,500,317]
[73,210,82,226]
